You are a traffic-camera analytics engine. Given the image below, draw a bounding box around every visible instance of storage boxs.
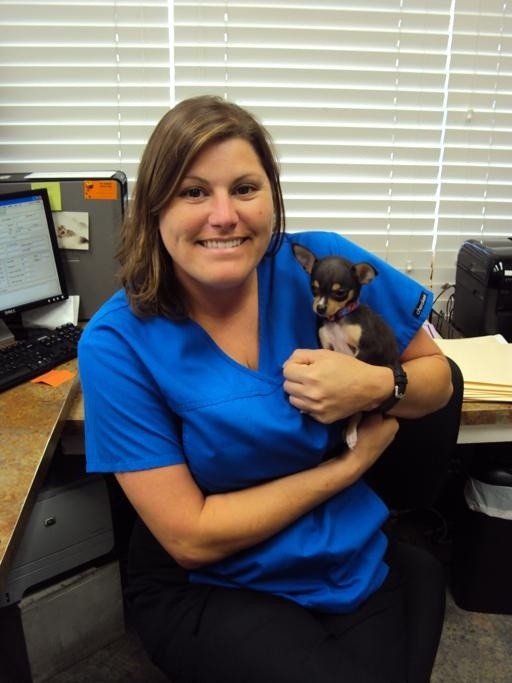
[18,561,127,683]
[2,473,117,607]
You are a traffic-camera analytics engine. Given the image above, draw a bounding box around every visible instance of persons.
[78,95,453,682]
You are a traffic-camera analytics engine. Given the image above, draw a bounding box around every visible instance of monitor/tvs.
[0,188,70,340]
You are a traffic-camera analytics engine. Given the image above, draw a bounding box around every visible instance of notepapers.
[31,370,76,388]
[30,182,61,210]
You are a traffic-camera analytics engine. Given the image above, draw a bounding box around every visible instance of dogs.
[290,240,404,456]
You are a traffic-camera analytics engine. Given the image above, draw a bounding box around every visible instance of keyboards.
[1,322,85,393]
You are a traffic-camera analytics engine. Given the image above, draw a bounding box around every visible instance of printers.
[454,240,512,345]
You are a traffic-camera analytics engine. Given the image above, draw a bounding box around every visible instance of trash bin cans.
[466,469,510,607]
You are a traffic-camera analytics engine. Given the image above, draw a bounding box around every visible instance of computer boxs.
[1,171,127,322]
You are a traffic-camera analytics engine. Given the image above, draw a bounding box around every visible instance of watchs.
[369,361,407,416]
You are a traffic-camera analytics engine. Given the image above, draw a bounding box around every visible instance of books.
[433,333,512,403]
[465,478,512,521]
[21,294,81,330]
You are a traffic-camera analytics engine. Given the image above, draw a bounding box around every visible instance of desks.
[0,319,90,570]
[61,378,512,448]
